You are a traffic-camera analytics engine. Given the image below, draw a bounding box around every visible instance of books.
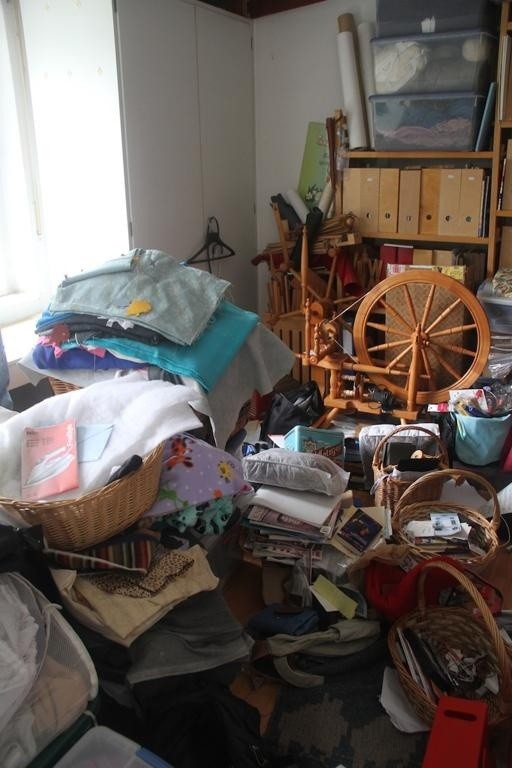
[240,485,472,709]
[19,418,80,502]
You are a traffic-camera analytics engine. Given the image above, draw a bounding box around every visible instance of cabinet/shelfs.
[334,150,497,390]
[487,1,512,281]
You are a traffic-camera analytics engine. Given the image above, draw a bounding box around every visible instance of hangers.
[186,216,235,264]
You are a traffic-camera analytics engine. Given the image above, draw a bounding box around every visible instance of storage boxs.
[476,280,512,335]
[376,0,503,36]
[368,92,488,151]
[53,723,175,768]
[25,693,102,768]
[370,26,498,93]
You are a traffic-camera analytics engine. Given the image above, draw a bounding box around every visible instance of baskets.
[391,469,500,580]
[373,426,450,512]
[388,560,511,728]
[1,376,168,551]
[0,376,250,552]
[372,425,512,728]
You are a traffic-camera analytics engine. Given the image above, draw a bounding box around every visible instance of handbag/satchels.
[260,380,327,448]
